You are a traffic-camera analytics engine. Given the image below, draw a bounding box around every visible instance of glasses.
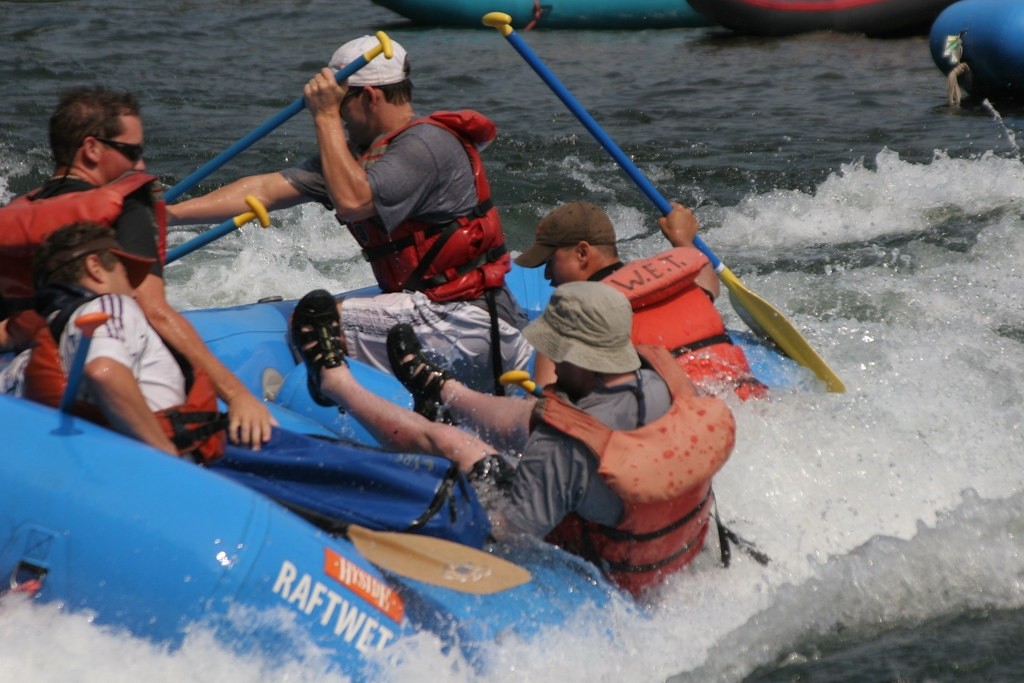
[76,134,145,162]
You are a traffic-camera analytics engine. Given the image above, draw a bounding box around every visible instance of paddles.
[164,195,272,267]
[161,28,394,204]
[499,366,547,398]
[226,472,534,596]
[486,9,848,394]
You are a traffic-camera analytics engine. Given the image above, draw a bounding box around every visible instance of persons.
[515,202,767,401]
[1,85,279,450]
[166,34,531,393]
[293,280,736,592]
[1,220,225,468]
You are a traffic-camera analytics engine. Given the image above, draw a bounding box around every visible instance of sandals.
[287,290,351,414]
[386,323,455,425]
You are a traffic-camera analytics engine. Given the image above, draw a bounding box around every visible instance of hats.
[522,280,642,373]
[327,34,408,87]
[514,201,617,268]
[41,238,158,289]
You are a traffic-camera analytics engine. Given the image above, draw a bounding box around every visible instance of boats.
[375,0,696,29]
[0,247,826,683]
[686,0,951,41]
[930,0,1024,109]
